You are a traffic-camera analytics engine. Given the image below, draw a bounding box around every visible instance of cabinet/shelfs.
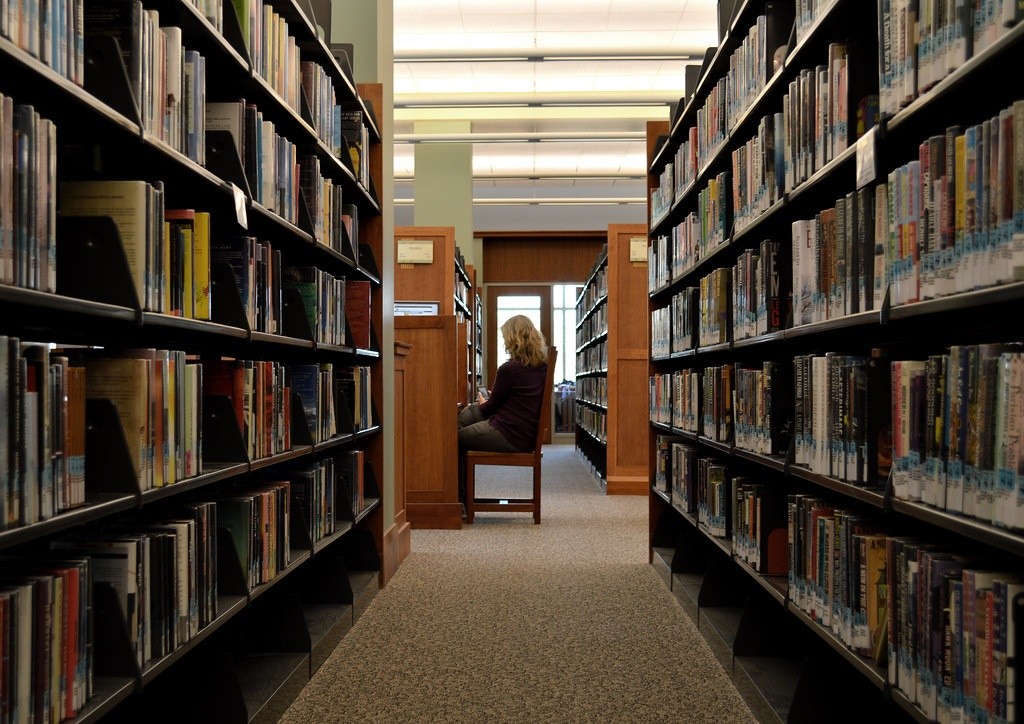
[393,225,483,531]
[575,223,649,496]
[0,1,384,724]
[645,0,1024,724]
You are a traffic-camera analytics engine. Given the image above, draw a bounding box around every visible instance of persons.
[456,314,547,518]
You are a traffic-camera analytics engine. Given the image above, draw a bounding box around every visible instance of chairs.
[467,346,558,525]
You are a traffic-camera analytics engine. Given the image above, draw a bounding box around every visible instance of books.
[574,265,607,446]
[454,241,482,403]
[0,0,378,724]
[646,0,1022,724]
